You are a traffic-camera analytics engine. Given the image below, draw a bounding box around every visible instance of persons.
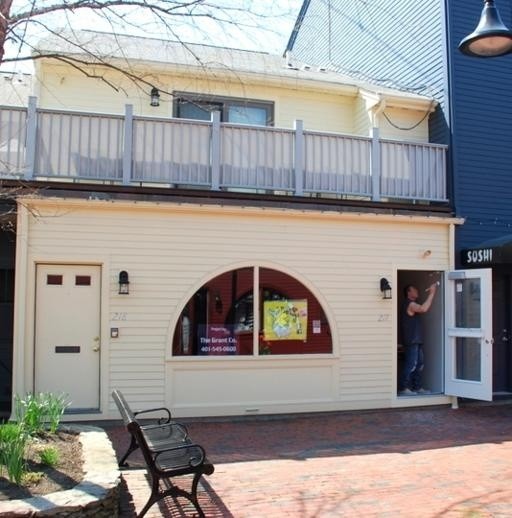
[399,283,436,395]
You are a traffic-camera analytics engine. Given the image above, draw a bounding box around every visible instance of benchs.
[108,387,214,517]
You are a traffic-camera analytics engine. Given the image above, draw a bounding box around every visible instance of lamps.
[118,271,130,295]
[381,278,392,299]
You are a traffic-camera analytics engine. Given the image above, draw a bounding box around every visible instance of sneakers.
[399,388,431,395]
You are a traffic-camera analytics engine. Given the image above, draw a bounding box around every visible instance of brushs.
[425,281,440,292]
